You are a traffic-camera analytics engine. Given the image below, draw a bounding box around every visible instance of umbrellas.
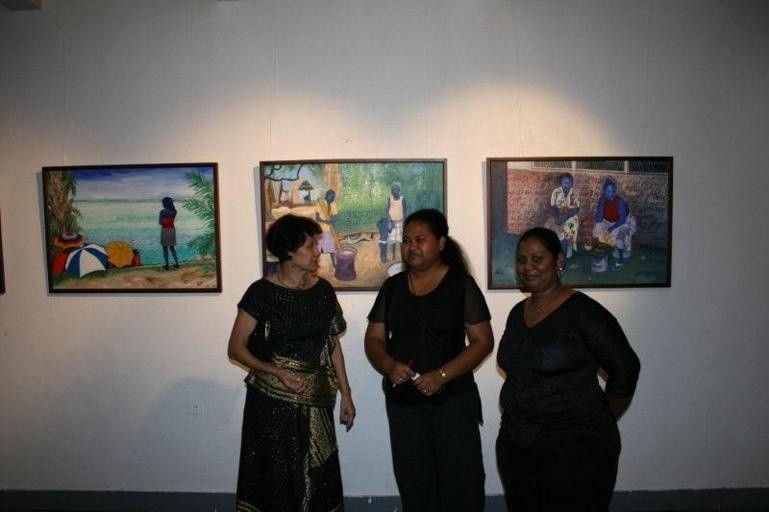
[54,231,134,281]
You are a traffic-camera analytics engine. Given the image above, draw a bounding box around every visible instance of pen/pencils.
[392,359,414,388]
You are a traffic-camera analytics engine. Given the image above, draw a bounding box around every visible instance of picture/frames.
[41,162,221,294]
[259,158,447,291]
[485,156,673,291]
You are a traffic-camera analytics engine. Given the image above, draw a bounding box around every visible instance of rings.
[399,378,401,381]
[425,389,427,393]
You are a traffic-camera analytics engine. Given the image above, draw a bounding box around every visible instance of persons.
[587,180,637,270]
[496,229,640,511]
[544,172,580,268]
[132,249,140,266]
[159,197,180,270]
[365,210,495,511]
[386,181,406,262]
[315,190,338,268]
[228,214,356,512]
[377,219,394,264]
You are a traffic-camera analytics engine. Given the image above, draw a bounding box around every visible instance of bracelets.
[437,369,449,383]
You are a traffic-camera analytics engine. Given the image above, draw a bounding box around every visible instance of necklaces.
[526,288,560,325]
[409,268,446,296]
[277,272,308,291]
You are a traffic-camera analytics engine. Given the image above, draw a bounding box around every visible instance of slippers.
[611,260,623,271]
